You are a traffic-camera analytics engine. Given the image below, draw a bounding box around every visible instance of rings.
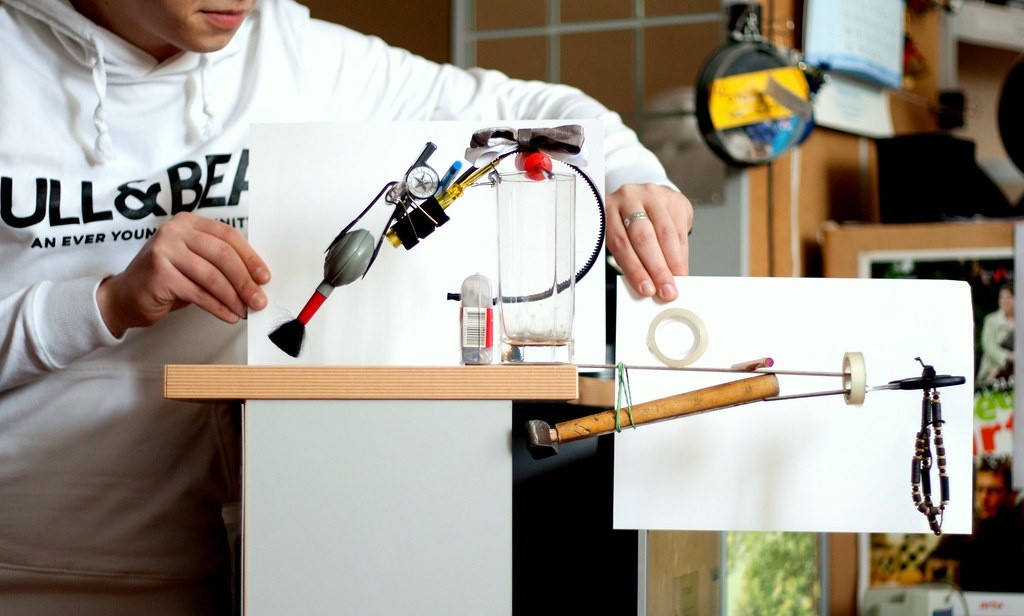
[624,211,649,228]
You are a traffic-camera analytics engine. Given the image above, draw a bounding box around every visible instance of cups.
[496,173,575,365]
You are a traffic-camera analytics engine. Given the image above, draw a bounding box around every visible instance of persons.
[0,0,694,616]
[922,455,1024,594]
[980,286,1015,388]
